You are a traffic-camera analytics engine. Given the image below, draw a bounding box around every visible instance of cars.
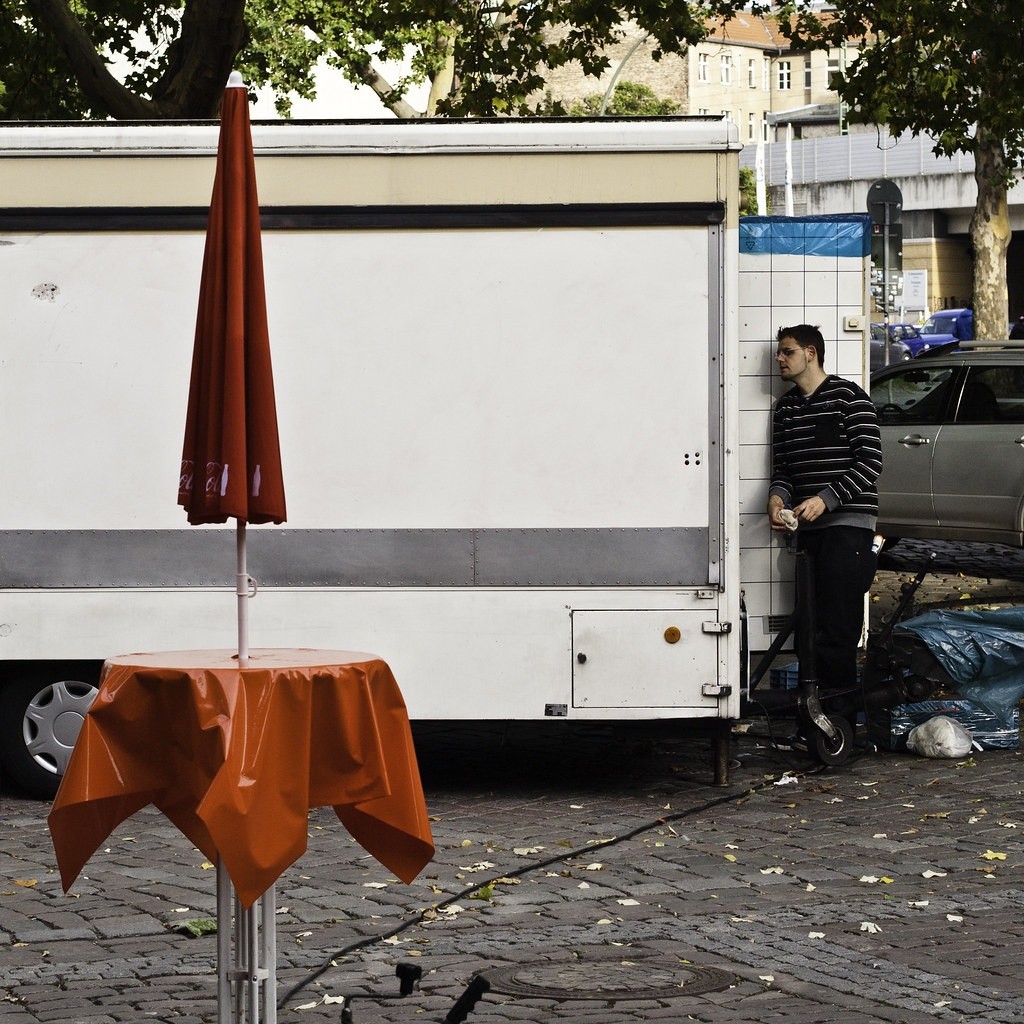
[868,307,977,373]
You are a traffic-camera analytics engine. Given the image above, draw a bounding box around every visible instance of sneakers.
[771,736,807,752]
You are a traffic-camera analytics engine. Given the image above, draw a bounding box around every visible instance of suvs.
[862,336,1024,553]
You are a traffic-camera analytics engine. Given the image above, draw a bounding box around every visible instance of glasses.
[774,347,806,359]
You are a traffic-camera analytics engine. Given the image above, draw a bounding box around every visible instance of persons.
[764,325,883,764]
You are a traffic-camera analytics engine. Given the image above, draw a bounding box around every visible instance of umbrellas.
[171,70,289,660]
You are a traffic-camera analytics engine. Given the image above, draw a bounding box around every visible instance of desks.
[46,650,434,1023]
